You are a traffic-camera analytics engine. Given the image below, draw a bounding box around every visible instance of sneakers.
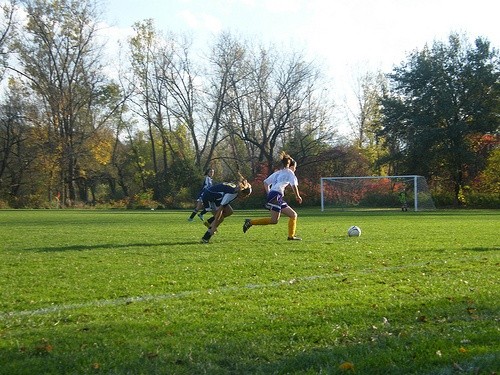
[243,218,251,233]
[287,236,302,240]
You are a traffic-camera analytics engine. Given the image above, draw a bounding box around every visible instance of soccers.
[347,225,361,237]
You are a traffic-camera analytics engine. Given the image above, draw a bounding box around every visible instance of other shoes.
[198,214,204,221]
[204,222,217,232]
[202,237,209,243]
[188,218,193,221]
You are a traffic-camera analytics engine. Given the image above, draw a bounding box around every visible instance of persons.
[398,186,408,211]
[243,150,302,241]
[187,168,214,222]
[200,178,252,244]
